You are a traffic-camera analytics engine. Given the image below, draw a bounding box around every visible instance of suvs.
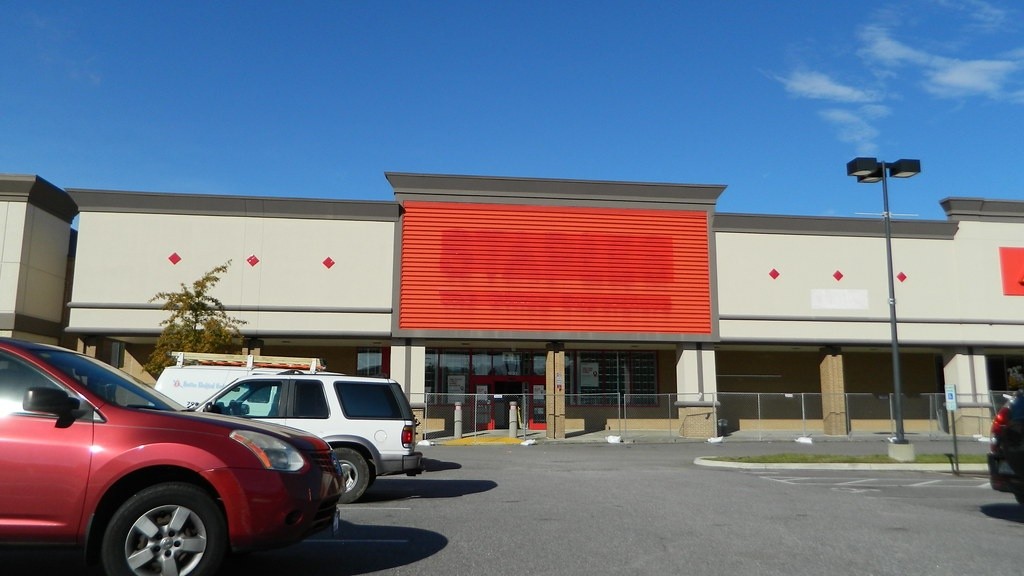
[191,368,425,505]
[0,335,347,576]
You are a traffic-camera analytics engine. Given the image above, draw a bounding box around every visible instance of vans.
[148,351,345,417]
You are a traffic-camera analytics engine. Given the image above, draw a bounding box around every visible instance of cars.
[985,392,1024,508]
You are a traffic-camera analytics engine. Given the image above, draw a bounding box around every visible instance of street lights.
[845,156,922,445]
